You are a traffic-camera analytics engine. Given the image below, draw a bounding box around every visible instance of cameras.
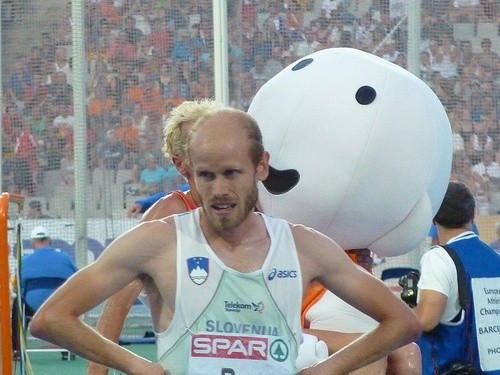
[399,271,420,307]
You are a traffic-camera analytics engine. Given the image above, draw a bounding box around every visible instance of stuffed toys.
[246,47,453,332]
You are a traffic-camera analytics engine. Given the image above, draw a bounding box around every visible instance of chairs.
[255,0,373,29]
[21,278,71,362]
[8,168,148,220]
[452,20,500,53]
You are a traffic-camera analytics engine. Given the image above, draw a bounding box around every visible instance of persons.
[0,0,500,219]
[28,108,426,375]
[11,225,80,362]
[411,181,500,375]
[87,98,422,375]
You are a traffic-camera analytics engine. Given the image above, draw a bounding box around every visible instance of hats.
[31,226,50,239]
